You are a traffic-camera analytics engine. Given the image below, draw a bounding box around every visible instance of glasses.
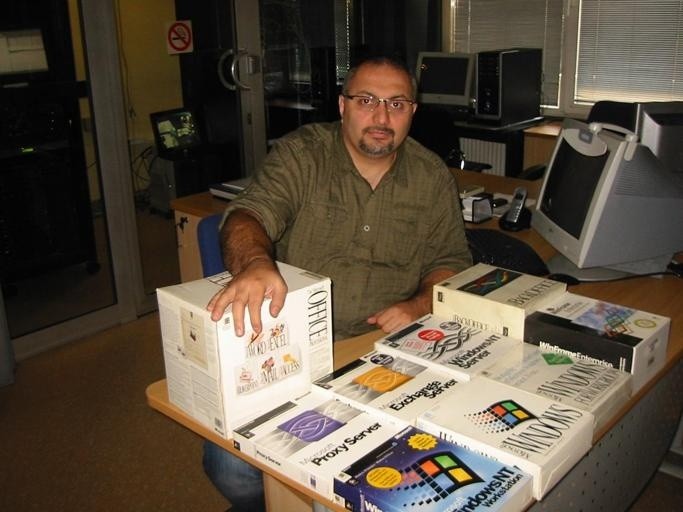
[340,91,416,114]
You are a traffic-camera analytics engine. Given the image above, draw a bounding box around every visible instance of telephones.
[498,184,530,231]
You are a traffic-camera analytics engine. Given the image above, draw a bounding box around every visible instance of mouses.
[548,273,579,286]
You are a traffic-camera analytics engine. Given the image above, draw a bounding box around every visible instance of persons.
[203,54,472,512]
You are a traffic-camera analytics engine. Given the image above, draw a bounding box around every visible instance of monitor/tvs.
[415,52,475,106]
[530,117,682,282]
[634,101,683,169]
[150,107,205,151]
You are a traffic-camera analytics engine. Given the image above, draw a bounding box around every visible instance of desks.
[146,120,681,512]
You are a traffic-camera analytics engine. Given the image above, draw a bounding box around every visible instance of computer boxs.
[474,48,542,125]
